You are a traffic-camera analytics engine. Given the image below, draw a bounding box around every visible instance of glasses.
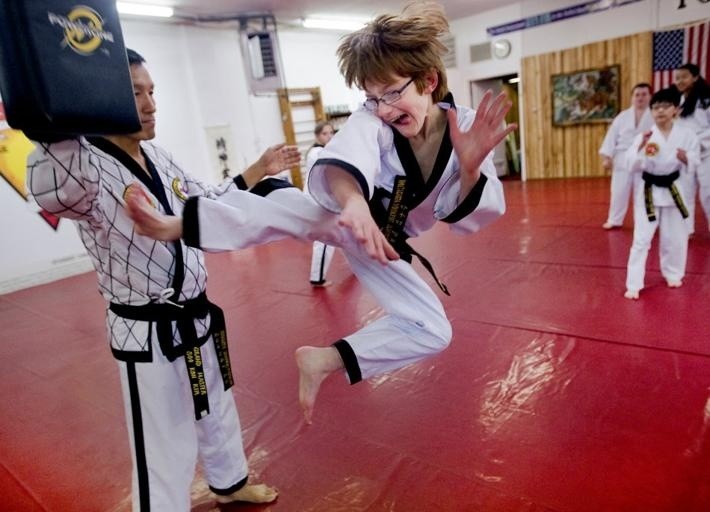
[363,77,415,112]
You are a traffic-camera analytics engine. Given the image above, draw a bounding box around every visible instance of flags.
[651,19,709,101]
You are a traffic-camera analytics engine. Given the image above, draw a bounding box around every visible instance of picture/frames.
[551,64,622,128]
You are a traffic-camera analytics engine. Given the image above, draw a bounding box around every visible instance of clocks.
[494,38,511,58]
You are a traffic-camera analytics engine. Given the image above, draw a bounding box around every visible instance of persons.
[623,89,701,300]
[302,119,340,286]
[597,82,654,229]
[122,0,520,426]
[672,62,710,242]
[26,49,303,511]
